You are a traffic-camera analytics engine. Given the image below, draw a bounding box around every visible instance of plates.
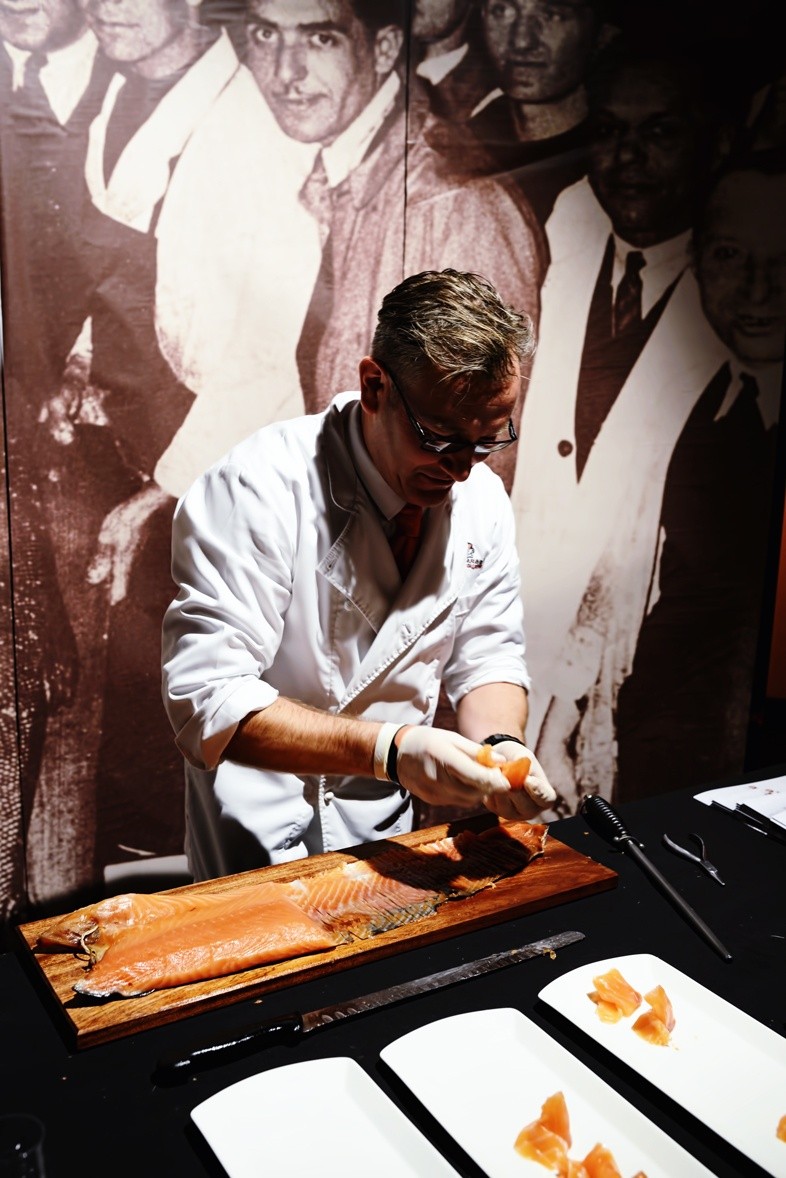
[378,1005,722,1178]
[536,950,786,1177]
[190,1055,466,1177]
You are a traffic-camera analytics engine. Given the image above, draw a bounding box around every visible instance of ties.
[298,150,334,245]
[717,372,768,435]
[614,251,648,334]
[21,51,56,124]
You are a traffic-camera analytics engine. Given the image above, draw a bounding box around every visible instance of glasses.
[385,368,517,456]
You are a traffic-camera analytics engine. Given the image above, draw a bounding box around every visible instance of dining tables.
[0,764,786,1178]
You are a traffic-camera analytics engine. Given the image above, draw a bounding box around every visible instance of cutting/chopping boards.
[18,804,621,1051]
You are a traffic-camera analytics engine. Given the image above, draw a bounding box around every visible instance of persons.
[617,148,786,811]
[0,0,606,772]
[510,37,731,821]
[160,266,557,880]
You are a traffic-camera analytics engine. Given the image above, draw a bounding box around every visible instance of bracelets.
[481,734,526,747]
[370,718,409,784]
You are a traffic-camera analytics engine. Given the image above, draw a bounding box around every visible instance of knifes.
[174,925,582,1079]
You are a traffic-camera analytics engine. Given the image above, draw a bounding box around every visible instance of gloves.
[482,740,556,822]
[374,722,510,810]
